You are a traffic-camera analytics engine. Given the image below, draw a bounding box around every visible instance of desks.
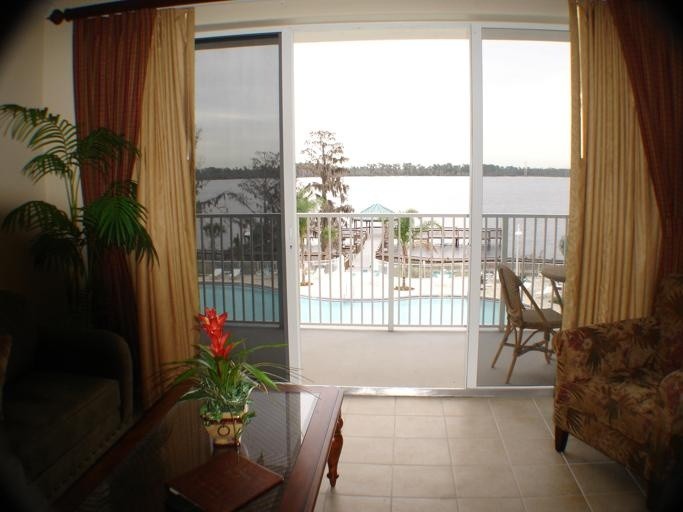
[540,264,566,358]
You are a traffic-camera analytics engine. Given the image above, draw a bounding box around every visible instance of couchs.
[0,287,136,511]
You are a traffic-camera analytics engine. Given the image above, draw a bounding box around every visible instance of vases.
[200,408,246,446]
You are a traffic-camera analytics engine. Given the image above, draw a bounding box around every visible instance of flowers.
[148,305,322,444]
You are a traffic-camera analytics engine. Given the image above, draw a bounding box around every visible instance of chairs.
[209,268,222,280]
[490,264,562,386]
[553,273,683,512]
[229,268,241,281]
